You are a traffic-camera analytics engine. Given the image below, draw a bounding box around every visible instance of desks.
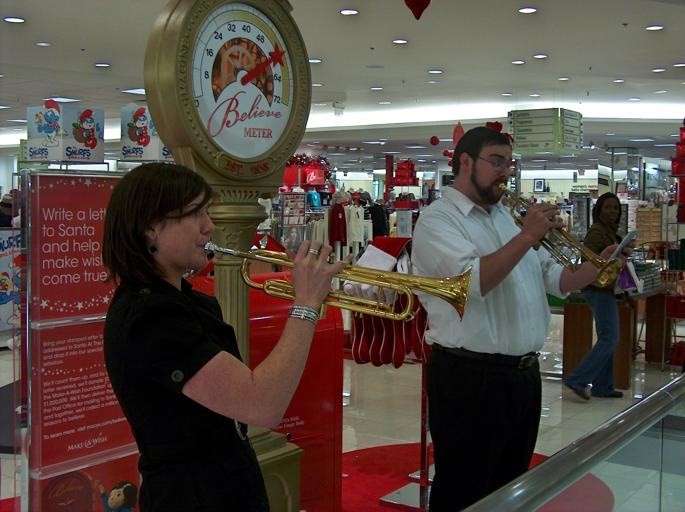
[562,286,672,389]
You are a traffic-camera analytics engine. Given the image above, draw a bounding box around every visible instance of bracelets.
[289,305,321,326]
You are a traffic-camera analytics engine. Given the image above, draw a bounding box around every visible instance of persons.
[557,191,624,401]
[99,161,355,511]
[331,185,372,206]
[411,124,635,512]
[283,227,301,253]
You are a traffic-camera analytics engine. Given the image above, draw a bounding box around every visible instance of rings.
[307,248,320,256]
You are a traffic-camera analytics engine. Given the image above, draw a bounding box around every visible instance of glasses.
[467,151,513,171]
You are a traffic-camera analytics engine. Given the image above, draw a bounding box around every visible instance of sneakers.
[565,379,625,400]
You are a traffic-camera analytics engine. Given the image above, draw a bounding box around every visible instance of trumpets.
[203,242,472,322]
[498,182,628,286]
